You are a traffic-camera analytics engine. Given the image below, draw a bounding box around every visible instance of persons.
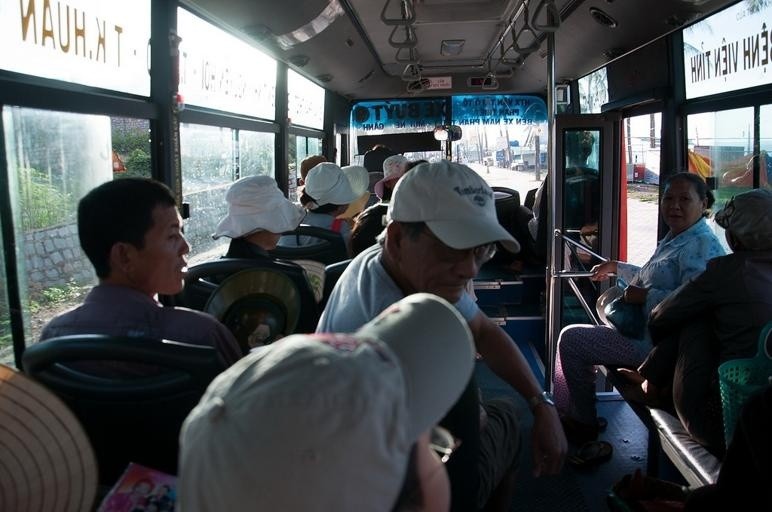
[615,189,772,447]
[517,129,599,271]
[557,173,728,456]
[40,177,242,376]
[283,143,438,258]
[608,313,772,511]
[213,175,307,290]
[316,157,572,512]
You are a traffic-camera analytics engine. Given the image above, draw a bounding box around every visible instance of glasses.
[419,238,497,263]
[427,425,454,464]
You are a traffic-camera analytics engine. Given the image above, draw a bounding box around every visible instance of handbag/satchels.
[595,279,644,340]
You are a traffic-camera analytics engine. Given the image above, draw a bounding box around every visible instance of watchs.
[529,391,557,412]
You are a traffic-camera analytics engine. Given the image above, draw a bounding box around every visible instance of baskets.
[718,321,772,451]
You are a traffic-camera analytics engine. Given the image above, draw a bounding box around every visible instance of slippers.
[565,417,613,466]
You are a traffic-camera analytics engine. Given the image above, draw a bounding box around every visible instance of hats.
[211,175,306,239]
[304,162,370,210]
[715,188,772,250]
[374,155,521,255]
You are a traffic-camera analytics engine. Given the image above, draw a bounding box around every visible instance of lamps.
[441,40,464,56]
